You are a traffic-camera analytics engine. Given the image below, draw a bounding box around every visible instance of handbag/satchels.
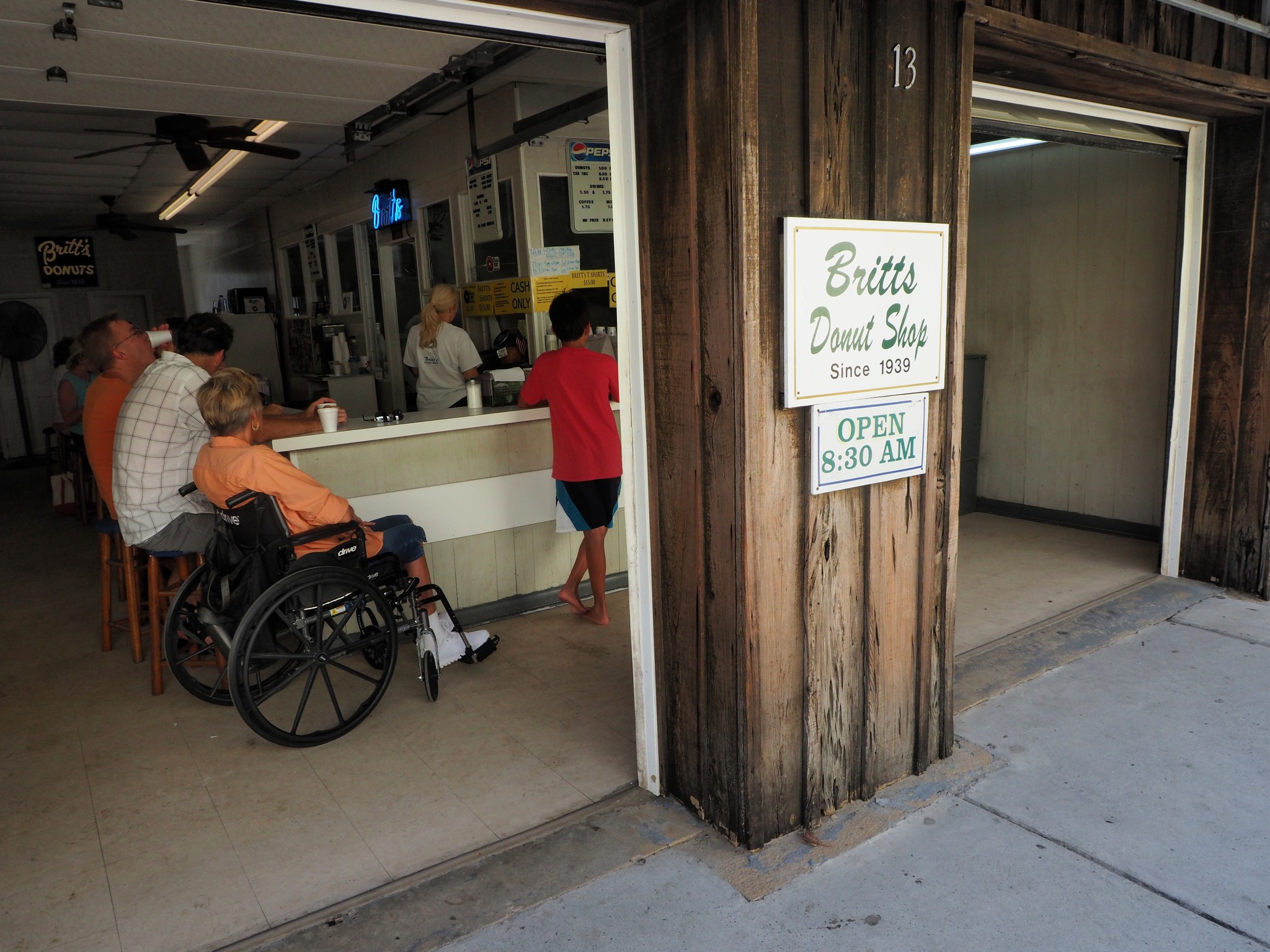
[50,472,76,518]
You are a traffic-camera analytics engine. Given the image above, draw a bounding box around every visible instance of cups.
[588,327,593,335]
[607,327,617,337]
[317,403,337,409]
[595,326,606,335]
[145,330,172,349]
[465,377,483,409]
[359,355,370,372]
[544,335,558,352]
[332,332,351,377]
[317,408,338,433]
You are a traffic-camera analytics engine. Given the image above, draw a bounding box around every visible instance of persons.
[49,337,83,520]
[191,367,489,671]
[518,291,622,626]
[57,339,104,520]
[76,315,284,533]
[402,283,484,412]
[112,310,348,632]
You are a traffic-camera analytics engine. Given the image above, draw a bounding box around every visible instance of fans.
[73,111,301,171]
[0,301,59,469]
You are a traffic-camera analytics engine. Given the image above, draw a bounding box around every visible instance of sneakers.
[425,620,490,668]
[412,610,455,634]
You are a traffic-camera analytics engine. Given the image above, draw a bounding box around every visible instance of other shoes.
[181,615,217,656]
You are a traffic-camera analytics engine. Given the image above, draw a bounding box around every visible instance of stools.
[43,427,228,696]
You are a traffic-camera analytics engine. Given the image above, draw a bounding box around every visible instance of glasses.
[113,326,144,351]
[221,353,226,362]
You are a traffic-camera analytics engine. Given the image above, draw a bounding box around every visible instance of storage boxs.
[227,288,269,315]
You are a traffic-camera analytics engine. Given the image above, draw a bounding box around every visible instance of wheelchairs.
[161,478,503,751]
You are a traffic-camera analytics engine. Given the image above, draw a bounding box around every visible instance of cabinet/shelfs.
[302,374,378,418]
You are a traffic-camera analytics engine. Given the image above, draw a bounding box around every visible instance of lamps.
[51,195,187,241]
[158,120,290,220]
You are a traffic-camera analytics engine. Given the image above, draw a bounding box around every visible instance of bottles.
[376,323,387,361]
[216,295,227,315]
